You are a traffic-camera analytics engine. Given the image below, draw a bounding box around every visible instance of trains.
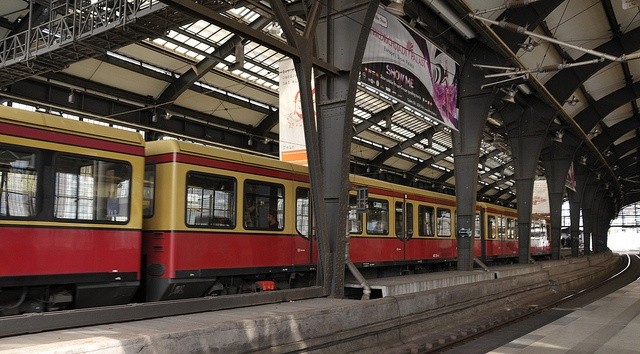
[0,106,552,318]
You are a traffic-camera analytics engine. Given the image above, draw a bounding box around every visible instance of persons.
[265,212,279,230]
